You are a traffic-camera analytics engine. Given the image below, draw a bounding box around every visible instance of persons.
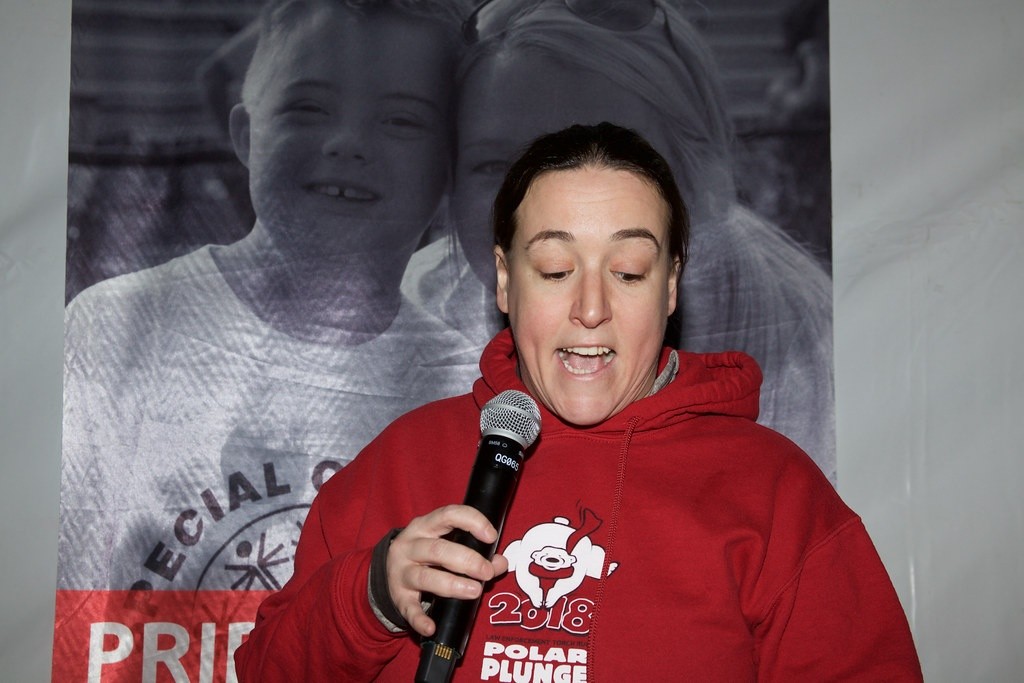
[53,0,489,682]
[231,115,924,682]
[394,0,838,487]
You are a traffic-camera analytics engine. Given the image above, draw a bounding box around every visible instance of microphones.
[414,389,543,683]
